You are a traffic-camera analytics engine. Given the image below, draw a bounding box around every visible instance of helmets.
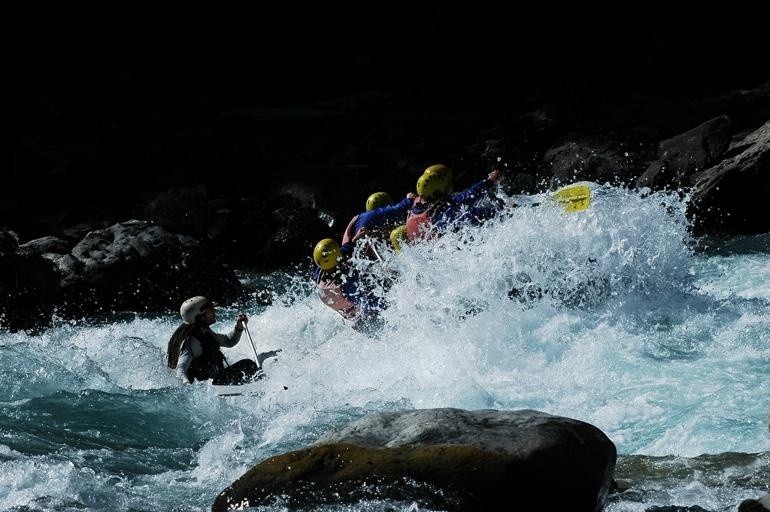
[179,296,209,324]
[366,192,392,211]
[313,238,341,270]
[416,164,453,197]
[390,225,407,251]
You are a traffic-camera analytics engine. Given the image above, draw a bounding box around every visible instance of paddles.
[502,184,591,213]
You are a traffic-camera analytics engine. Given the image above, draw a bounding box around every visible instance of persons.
[310,157,514,333]
[166,294,261,387]
